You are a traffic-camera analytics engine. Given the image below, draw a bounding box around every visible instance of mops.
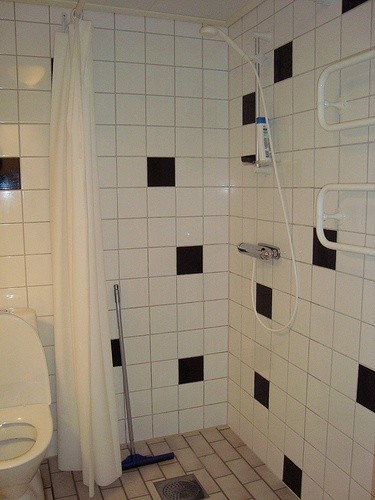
[113,285,175,472]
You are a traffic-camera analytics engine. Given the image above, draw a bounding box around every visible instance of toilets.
[0,307,55,500]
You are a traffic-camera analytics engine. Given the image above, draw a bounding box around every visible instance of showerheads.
[200,26,251,67]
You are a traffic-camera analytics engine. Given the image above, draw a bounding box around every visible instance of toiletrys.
[256,117,271,160]
